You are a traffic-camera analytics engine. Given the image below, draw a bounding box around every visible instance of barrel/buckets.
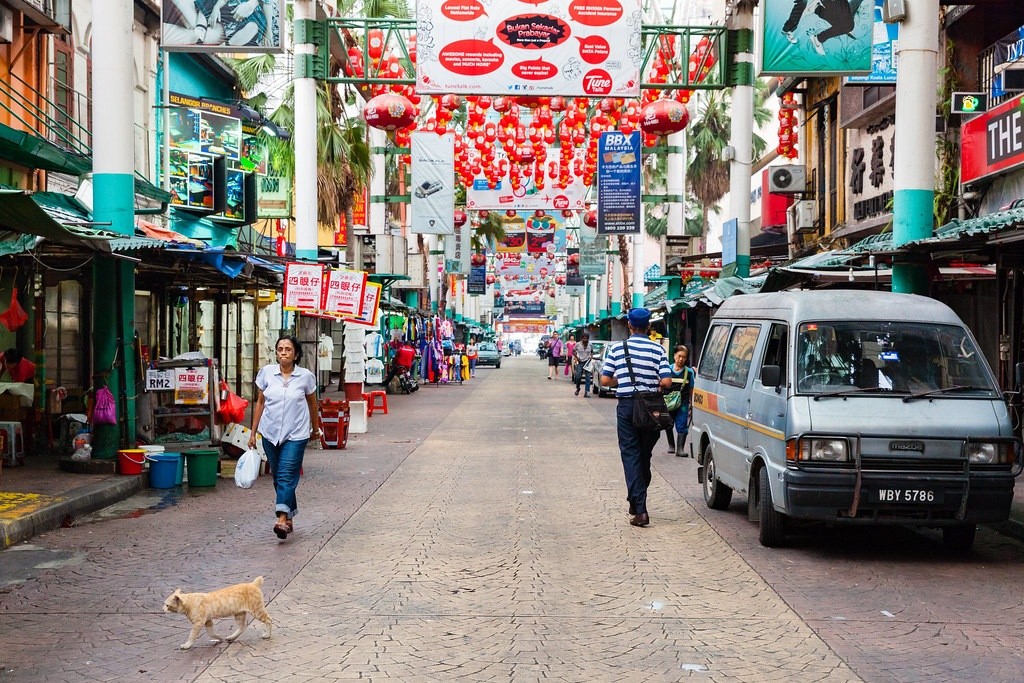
[144,452,185,488]
[185,450,218,486]
[139,445,165,472]
[118,449,147,475]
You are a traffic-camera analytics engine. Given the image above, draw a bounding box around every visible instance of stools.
[0,421,24,468]
[319,391,388,450]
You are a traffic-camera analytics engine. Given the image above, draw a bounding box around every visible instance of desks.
[0,382,81,448]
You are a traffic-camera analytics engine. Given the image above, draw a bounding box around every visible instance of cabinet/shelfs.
[147,358,215,447]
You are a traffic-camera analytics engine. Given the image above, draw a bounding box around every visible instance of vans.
[689,287,1024,549]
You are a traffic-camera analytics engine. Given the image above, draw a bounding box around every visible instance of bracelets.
[312,428,320,434]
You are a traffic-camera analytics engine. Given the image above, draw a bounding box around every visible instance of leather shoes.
[630,512,650,525]
[628,506,636,514]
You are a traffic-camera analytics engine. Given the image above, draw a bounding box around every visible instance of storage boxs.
[220,422,267,478]
[342,400,369,434]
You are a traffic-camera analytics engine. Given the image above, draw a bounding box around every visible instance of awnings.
[0,191,498,342]
[562,202,1023,337]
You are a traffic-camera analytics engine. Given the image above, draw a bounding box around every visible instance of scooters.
[396,365,420,394]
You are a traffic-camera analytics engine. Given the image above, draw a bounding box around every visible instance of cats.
[162,575,272,650]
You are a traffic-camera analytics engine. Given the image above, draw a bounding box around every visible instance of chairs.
[777,333,814,386]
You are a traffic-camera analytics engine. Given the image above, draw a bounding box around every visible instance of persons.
[509,341,518,356]
[496,336,503,350]
[601,308,672,526]
[781,0,854,54]
[664,345,694,458]
[648,327,662,341]
[247,336,324,540]
[466,337,479,378]
[544,331,563,380]
[0,348,36,457]
[572,334,593,398]
[564,335,577,375]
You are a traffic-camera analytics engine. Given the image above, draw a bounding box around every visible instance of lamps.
[153,97,290,140]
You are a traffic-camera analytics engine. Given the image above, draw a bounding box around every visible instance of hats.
[628,307,650,326]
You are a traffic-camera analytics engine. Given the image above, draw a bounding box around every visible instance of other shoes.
[555,375,559,380]
[548,376,551,380]
[576,388,580,395]
[584,393,591,397]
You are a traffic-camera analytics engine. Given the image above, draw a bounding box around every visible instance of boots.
[675,433,688,455]
[666,429,675,453]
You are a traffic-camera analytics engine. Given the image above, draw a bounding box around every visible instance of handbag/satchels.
[235,447,260,489]
[663,390,682,410]
[92,386,117,425]
[0,287,28,332]
[548,348,553,356]
[632,392,672,429]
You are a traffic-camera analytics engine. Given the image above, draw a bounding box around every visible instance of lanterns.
[346,24,801,304]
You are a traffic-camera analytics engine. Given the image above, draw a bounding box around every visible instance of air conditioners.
[794,200,819,233]
[768,165,807,193]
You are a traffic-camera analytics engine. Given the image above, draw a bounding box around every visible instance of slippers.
[274,523,287,539]
[285,522,293,533]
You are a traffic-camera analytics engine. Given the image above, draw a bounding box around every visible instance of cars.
[473,341,502,368]
[589,339,622,398]
